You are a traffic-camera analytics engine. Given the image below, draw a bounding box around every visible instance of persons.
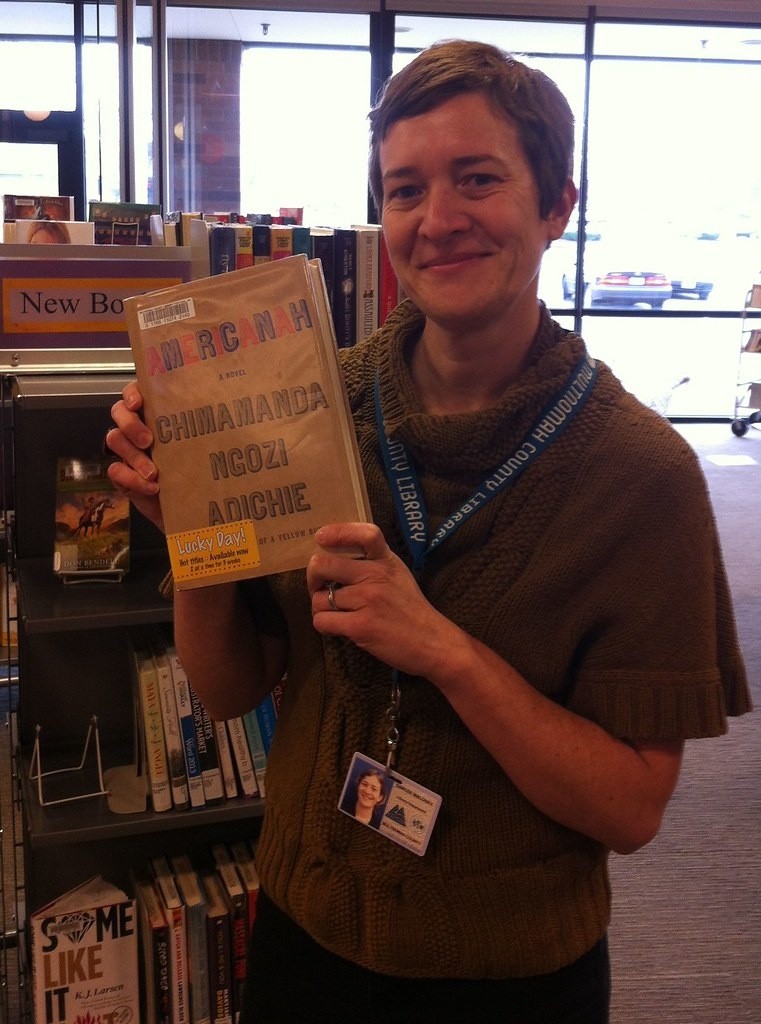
[339,767,388,831]
[103,35,755,1024]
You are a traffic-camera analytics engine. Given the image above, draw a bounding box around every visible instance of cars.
[662,258,711,300]
[589,260,672,309]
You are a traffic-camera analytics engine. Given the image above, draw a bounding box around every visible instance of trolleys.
[732,285,761,437]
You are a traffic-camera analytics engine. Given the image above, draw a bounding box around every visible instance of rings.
[326,582,343,612]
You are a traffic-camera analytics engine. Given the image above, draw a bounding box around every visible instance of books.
[122,253,376,591]
[2,194,408,348]
[30,816,259,1024]
[51,456,172,603]
[126,626,292,812]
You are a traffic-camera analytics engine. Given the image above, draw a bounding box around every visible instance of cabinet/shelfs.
[9,374,293,1023]
